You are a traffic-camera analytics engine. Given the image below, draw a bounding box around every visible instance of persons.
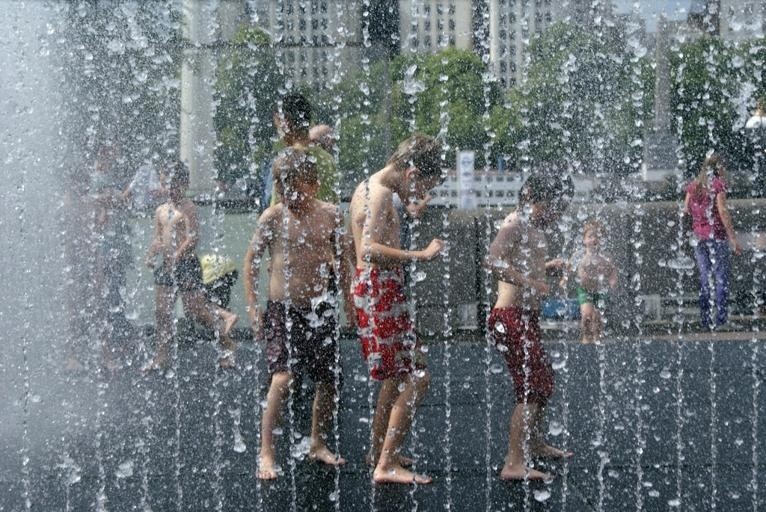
[680,151,744,332]
[241,149,358,480]
[345,130,454,485]
[55,104,238,372]
[261,95,341,297]
[563,224,616,347]
[478,167,574,481]
